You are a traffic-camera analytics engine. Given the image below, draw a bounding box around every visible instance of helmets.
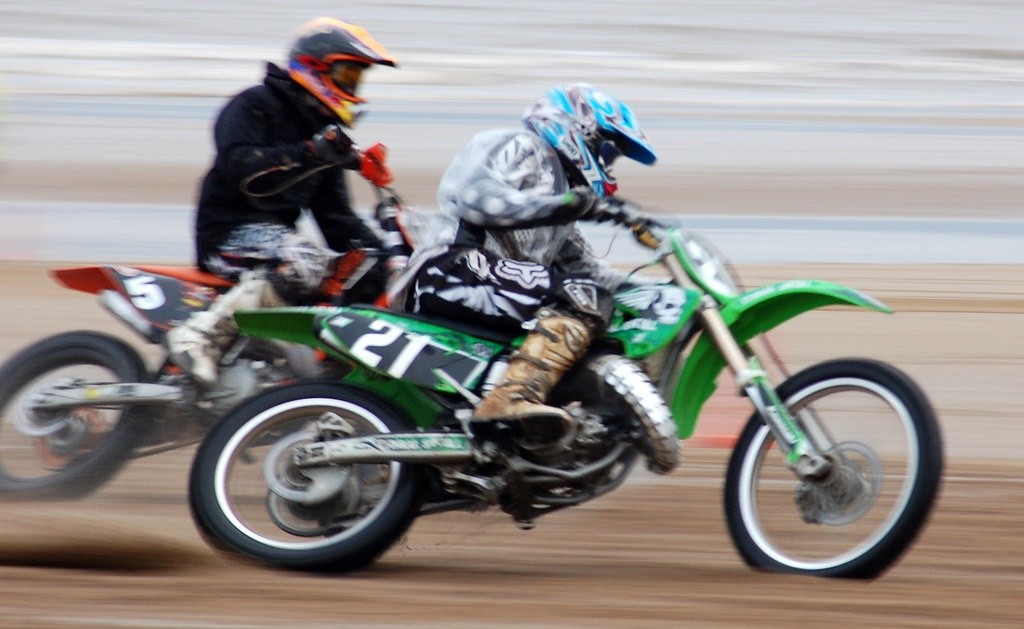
[523,84,658,202]
[288,16,399,129]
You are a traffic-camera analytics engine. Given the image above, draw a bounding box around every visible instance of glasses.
[327,60,361,93]
[598,139,621,173]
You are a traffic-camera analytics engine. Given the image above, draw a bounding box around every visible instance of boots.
[469,308,595,446]
[166,280,266,388]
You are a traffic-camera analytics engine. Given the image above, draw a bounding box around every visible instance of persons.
[387,83,673,445]
[165,17,396,387]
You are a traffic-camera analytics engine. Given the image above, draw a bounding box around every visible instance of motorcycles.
[0,124,448,499]
[183,203,949,592]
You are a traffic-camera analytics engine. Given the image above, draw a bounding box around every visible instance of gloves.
[312,124,353,161]
[567,183,601,218]
